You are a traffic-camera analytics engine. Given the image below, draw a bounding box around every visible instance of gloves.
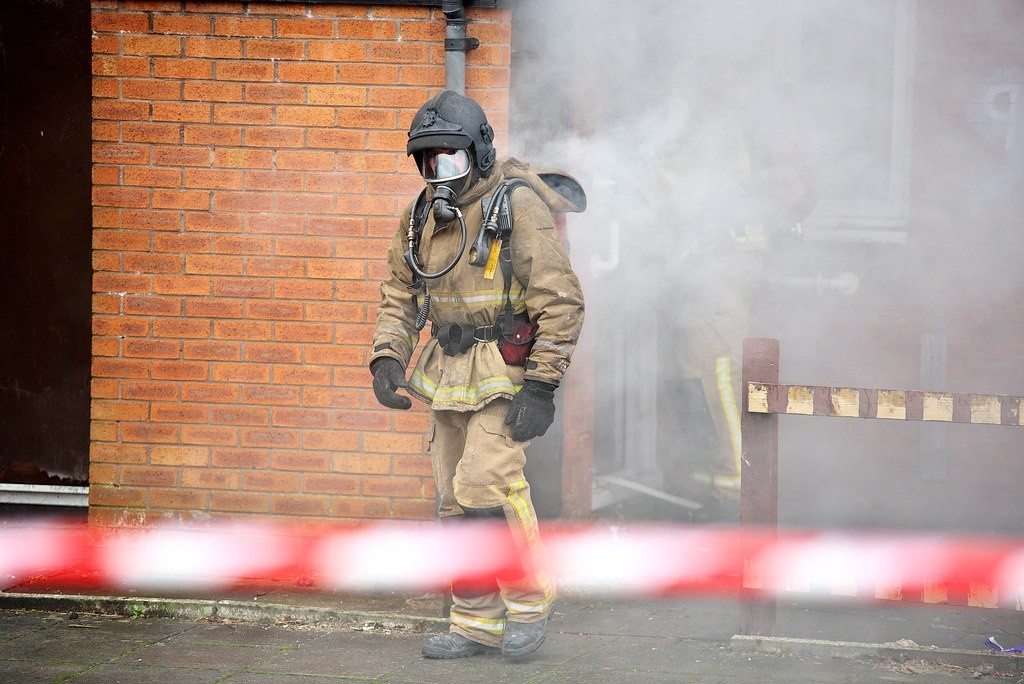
[371,358,412,410]
[505,380,555,442]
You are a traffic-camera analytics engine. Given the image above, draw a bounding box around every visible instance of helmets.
[406,89,496,178]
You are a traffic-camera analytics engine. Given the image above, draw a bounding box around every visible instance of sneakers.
[422,632,501,658]
[502,602,556,657]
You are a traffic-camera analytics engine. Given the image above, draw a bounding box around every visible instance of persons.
[370,92,588,662]
